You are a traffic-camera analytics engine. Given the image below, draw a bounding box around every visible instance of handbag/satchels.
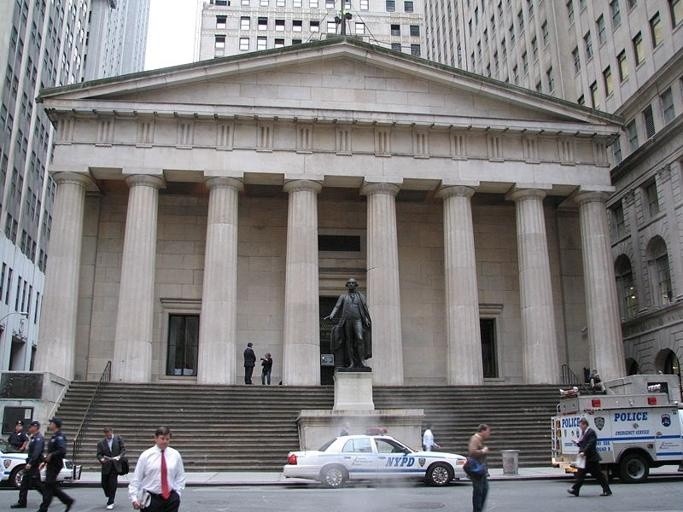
[113,457,129,475]
[463,457,488,481]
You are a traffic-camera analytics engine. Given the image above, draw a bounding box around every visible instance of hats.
[16,420,24,425]
[49,418,62,427]
[31,421,40,426]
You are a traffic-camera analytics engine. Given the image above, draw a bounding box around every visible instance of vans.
[549,373,682,482]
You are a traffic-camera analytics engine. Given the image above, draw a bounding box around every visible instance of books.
[574,453,586,470]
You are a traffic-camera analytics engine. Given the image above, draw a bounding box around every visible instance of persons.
[34,417,75,512]
[95,427,129,509]
[323,278,373,371]
[7,421,30,455]
[9,420,51,508]
[422,423,441,452]
[243,342,255,384]
[340,421,350,436]
[464,424,490,512]
[127,426,186,512]
[567,416,611,498]
[259,352,272,384]
[588,368,607,394]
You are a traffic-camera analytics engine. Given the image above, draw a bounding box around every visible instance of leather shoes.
[600,492,612,496]
[37,509,48,512]
[64,499,73,512]
[11,503,27,508]
[567,490,579,496]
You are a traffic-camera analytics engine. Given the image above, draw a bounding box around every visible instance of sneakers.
[105,497,109,502]
[106,503,114,510]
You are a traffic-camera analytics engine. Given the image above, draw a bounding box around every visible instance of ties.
[161,450,171,500]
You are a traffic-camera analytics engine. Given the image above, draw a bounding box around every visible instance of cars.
[284,434,469,487]
[0,442,82,489]
